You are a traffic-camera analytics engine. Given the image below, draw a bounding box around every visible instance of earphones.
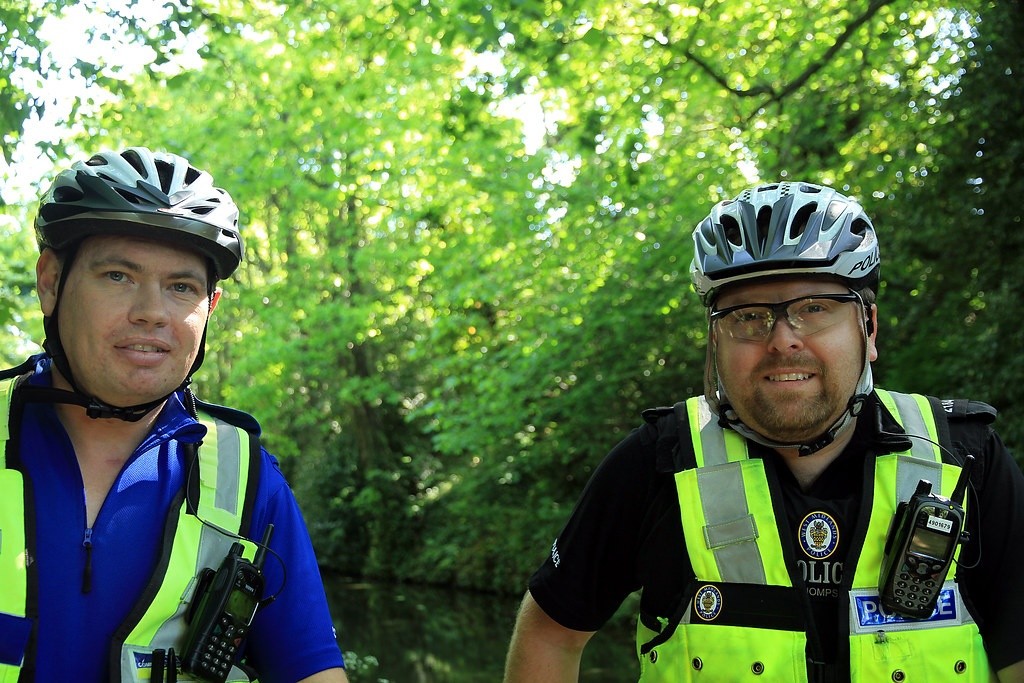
[866,306,874,336]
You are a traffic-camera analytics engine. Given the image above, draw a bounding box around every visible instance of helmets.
[689,178,880,306]
[32,145,244,292]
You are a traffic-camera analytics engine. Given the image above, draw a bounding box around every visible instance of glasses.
[709,293,860,343]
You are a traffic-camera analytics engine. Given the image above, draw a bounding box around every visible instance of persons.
[0,147,349,683]
[502,182,1024,683]
